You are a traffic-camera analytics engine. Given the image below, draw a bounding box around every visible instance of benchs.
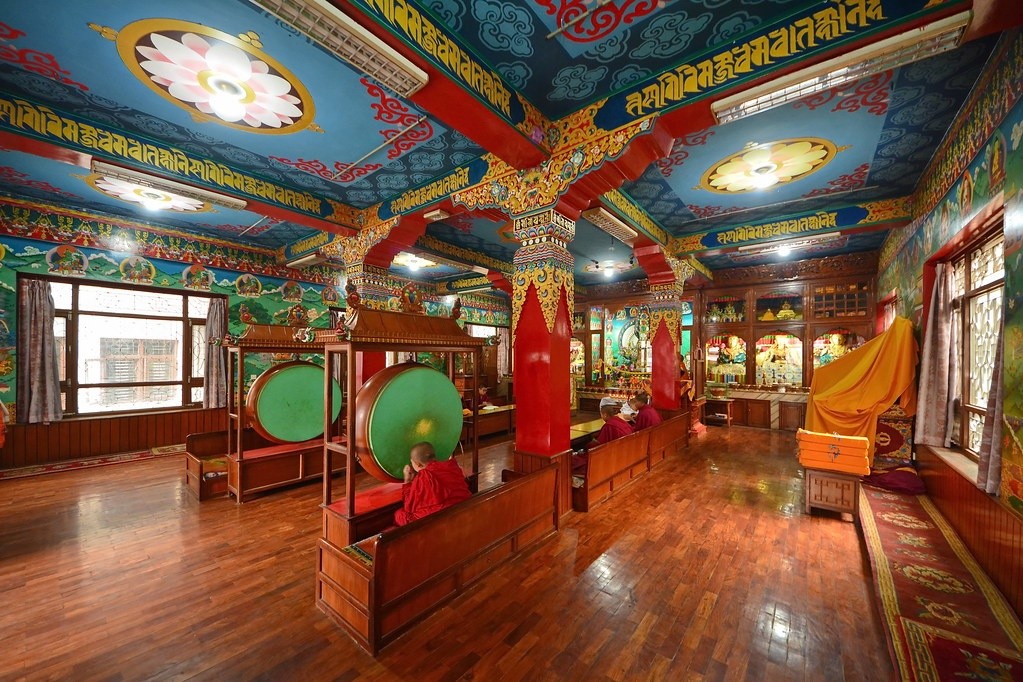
[184,428,279,502]
[573,412,690,512]
[489,395,507,407]
[314,461,561,657]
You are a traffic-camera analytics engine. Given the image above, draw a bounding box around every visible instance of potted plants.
[704,382,730,400]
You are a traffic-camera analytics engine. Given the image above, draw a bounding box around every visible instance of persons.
[710,336,747,373]
[390,440,475,529]
[584,405,633,450]
[754,334,801,372]
[811,333,853,370]
[623,394,666,433]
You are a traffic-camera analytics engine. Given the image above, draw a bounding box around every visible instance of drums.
[246,361,344,446]
[353,362,462,486]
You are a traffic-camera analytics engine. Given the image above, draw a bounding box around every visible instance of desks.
[570,428,590,446]
[469,407,510,446]
[459,416,468,446]
[570,418,606,434]
[500,404,516,433]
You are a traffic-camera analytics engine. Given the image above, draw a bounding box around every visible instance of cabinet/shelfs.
[706,399,734,426]
[781,402,805,431]
[728,398,770,428]
